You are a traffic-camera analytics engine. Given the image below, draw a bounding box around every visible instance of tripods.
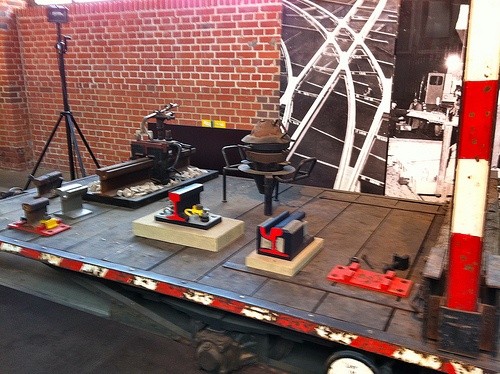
[23,24,102,190]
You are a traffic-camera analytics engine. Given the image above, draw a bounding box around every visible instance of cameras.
[47,5,68,23]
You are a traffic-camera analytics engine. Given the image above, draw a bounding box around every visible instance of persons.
[407,97,422,129]
